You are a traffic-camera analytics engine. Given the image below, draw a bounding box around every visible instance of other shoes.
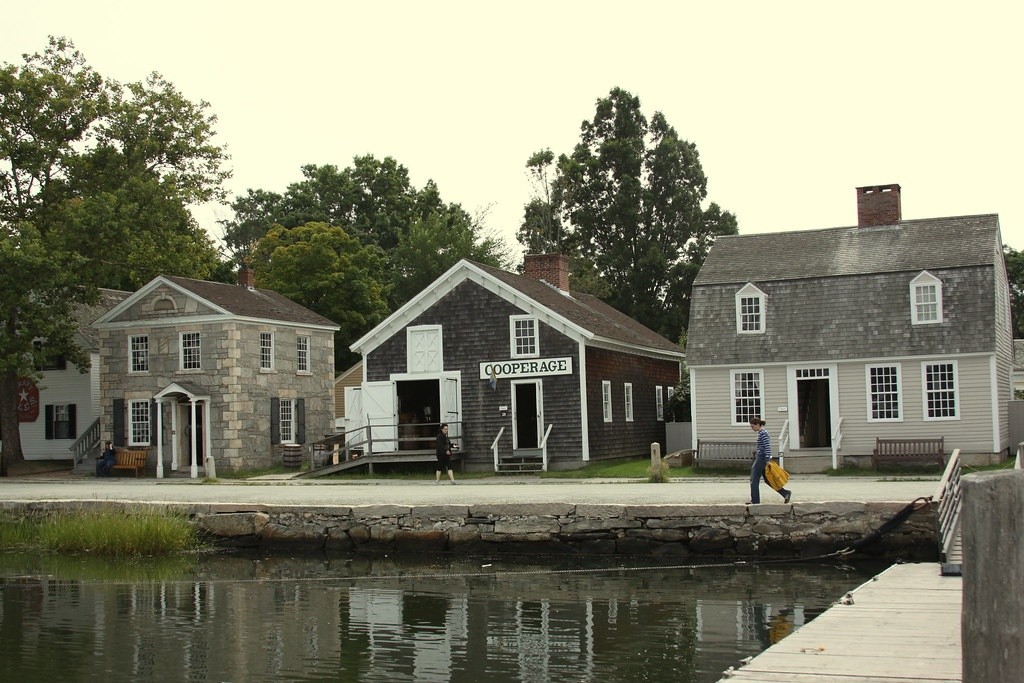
[745,501,757,504]
[435,481,441,486]
[784,491,791,503]
[450,480,460,485]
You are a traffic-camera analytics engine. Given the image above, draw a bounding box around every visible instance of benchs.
[691,439,757,471]
[873,437,944,473]
[97,449,147,479]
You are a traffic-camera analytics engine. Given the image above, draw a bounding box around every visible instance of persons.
[435,424,460,485]
[744,417,792,504]
[95,441,116,479]
